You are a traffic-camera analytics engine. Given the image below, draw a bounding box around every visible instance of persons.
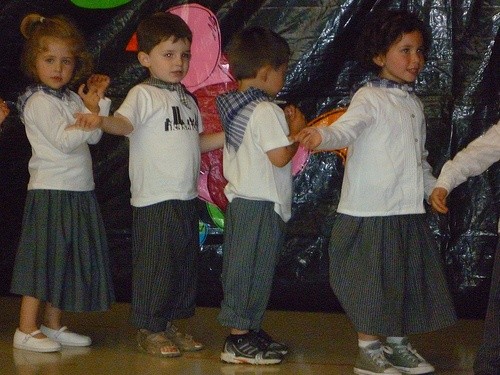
[0,98,10,126]
[288,14,463,375]
[216,27,314,364]
[65,12,226,355]
[430,120,500,375]
[11,13,112,352]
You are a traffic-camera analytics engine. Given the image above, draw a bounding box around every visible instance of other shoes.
[162,320,204,351]
[471,364,500,375]
[135,328,183,358]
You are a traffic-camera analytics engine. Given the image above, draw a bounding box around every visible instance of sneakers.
[383,336,435,375]
[40,324,91,346]
[353,341,402,375]
[12,328,62,353]
[219,327,288,365]
